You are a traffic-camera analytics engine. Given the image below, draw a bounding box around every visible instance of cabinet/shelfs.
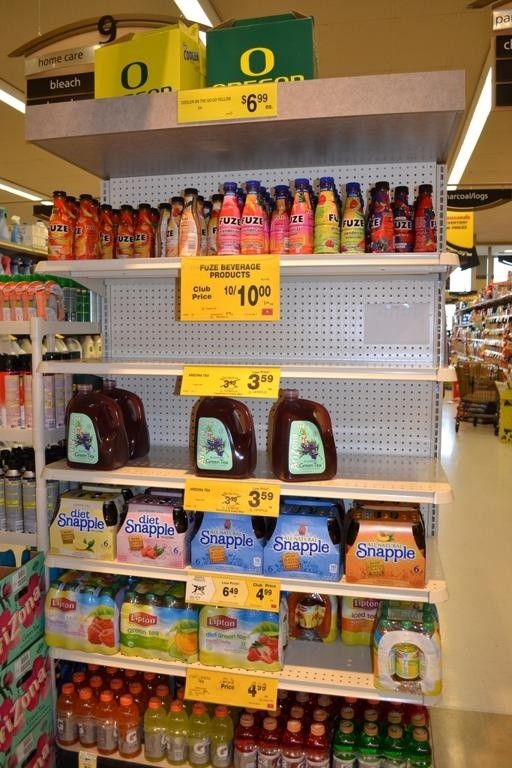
[449,294,512,373]
[0,241,462,767]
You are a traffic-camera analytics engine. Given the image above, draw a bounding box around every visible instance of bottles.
[0,250,91,321]
[47,177,438,261]
[0,468,39,536]
[0,205,46,249]
[52,658,435,768]
[2,335,105,428]
[45,566,444,700]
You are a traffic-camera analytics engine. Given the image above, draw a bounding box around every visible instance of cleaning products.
[0,204,11,241]
[11,213,23,242]
[0,335,101,359]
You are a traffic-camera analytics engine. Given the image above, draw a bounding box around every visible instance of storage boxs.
[25,12,319,106]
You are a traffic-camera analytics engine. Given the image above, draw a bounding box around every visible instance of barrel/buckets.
[266,388,337,482]
[101,378,148,459]
[64,379,131,470]
[188,397,257,478]
